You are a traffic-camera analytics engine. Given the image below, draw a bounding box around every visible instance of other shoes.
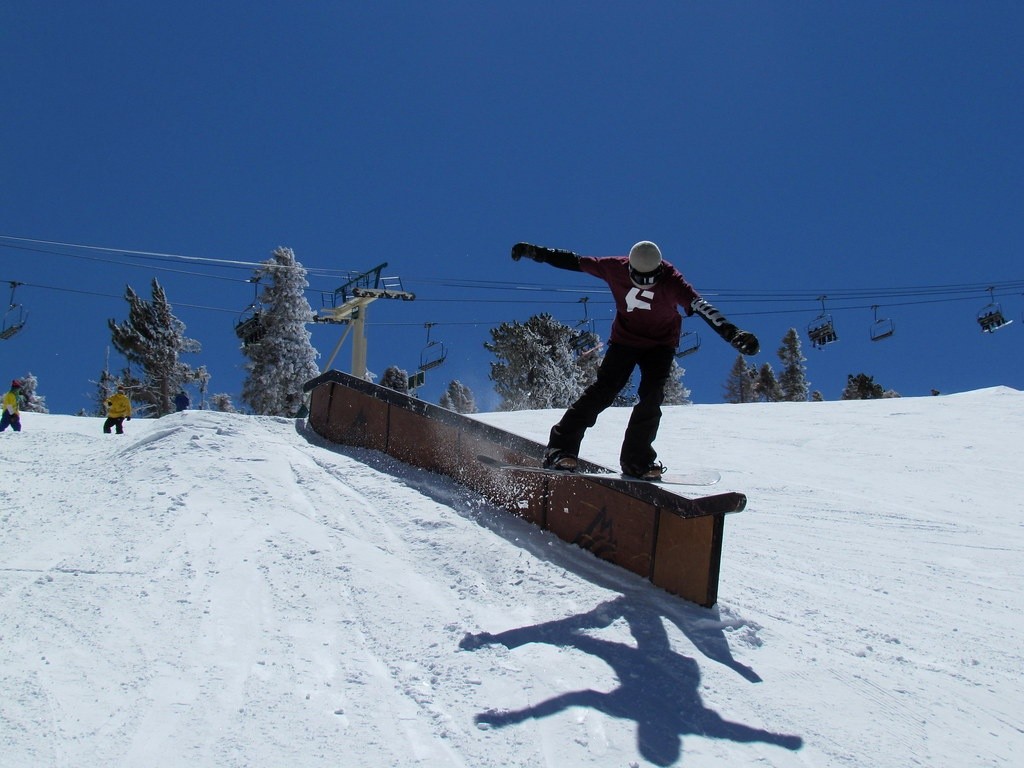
[542,448,577,470]
[619,454,661,479]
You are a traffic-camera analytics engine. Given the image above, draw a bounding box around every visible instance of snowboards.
[479,453,722,488]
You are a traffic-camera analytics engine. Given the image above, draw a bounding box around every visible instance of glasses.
[630,266,659,285]
[119,390,124,393]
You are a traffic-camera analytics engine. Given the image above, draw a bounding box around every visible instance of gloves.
[127,417,130,421]
[11,415,16,423]
[511,242,547,264]
[721,323,759,356]
[108,402,113,407]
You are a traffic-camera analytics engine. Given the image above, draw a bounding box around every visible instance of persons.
[0,380,22,432]
[173,391,189,412]
[511,240,759,479]
[978,310,1006,332]
[568,329,603,362]
[104,387,130,434]
[809,321,836,346]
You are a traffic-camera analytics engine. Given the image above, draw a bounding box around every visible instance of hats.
[118,386,124,390]
[12,380,21,388]
[629,241,662,273]
